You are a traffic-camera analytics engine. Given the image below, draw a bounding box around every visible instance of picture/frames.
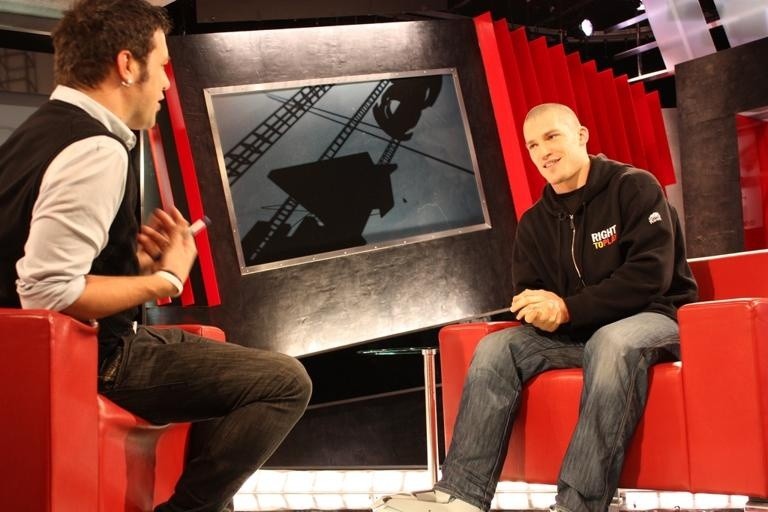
[202,67,493,276]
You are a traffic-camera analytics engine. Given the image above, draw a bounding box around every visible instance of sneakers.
[373,489,486,512]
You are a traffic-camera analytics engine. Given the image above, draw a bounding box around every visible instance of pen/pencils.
[154,216,212,261]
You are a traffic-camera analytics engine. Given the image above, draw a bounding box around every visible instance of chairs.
[0,308,227,512]
[437,245,768,509]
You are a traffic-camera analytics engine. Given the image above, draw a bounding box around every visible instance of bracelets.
[153,266,186,299]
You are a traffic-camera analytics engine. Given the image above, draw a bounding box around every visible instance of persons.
[369,102,700,512]
[0,0,315,511]
